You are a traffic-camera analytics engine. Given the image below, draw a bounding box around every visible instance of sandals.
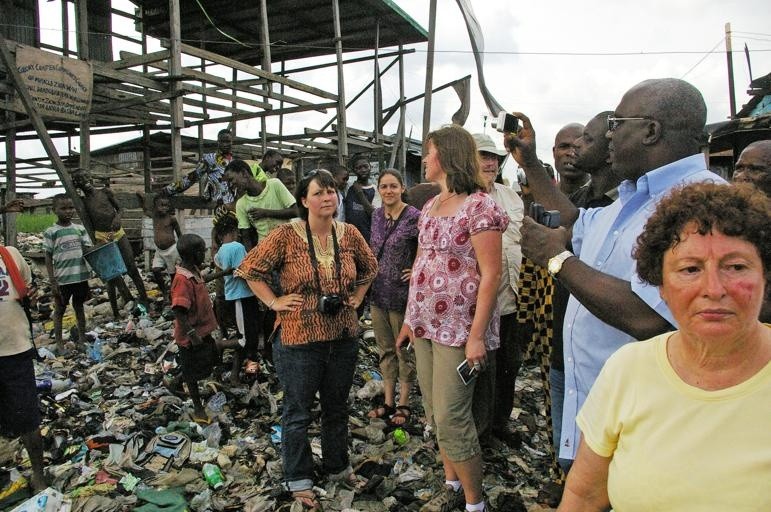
[367,403,394,422]
[389,406,411,427]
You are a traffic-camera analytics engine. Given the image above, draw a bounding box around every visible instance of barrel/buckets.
[84,242,128,284]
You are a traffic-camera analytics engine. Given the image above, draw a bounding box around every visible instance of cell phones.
[457,359,479,386]
[542,210,561,228]
[529,201,543,224]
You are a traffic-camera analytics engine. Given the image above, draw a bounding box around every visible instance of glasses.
[608,115,655,132]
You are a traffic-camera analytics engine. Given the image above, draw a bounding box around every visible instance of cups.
[370,417,386,434]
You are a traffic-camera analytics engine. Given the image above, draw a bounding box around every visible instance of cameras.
[491,111,519,133]
[321,293,340,314]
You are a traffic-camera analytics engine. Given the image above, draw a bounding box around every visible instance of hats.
[472,134,511,169]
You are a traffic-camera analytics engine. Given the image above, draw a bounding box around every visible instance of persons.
[472,110,619,469]
[556,179,771,512]
[155,129,298,375]
[1,241,50,500]
[44,193,110,358]
[169,234,234,427]
[398,126,508,512]
[135,191,183,302]
[732,138,771,327]
[234,169,380,509]
[326,156,444,426]
[504,76,732,477]
[72,169,151,320]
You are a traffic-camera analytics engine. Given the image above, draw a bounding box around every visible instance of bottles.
[95,336,101,358]
[36,379,69,392]
[202,462,225,489]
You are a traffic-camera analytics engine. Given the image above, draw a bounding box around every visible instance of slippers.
[189,410,214,430]
[290,488,323,510]
[342,473,367,492]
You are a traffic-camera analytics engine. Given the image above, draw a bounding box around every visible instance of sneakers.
[419,483,466,512]
